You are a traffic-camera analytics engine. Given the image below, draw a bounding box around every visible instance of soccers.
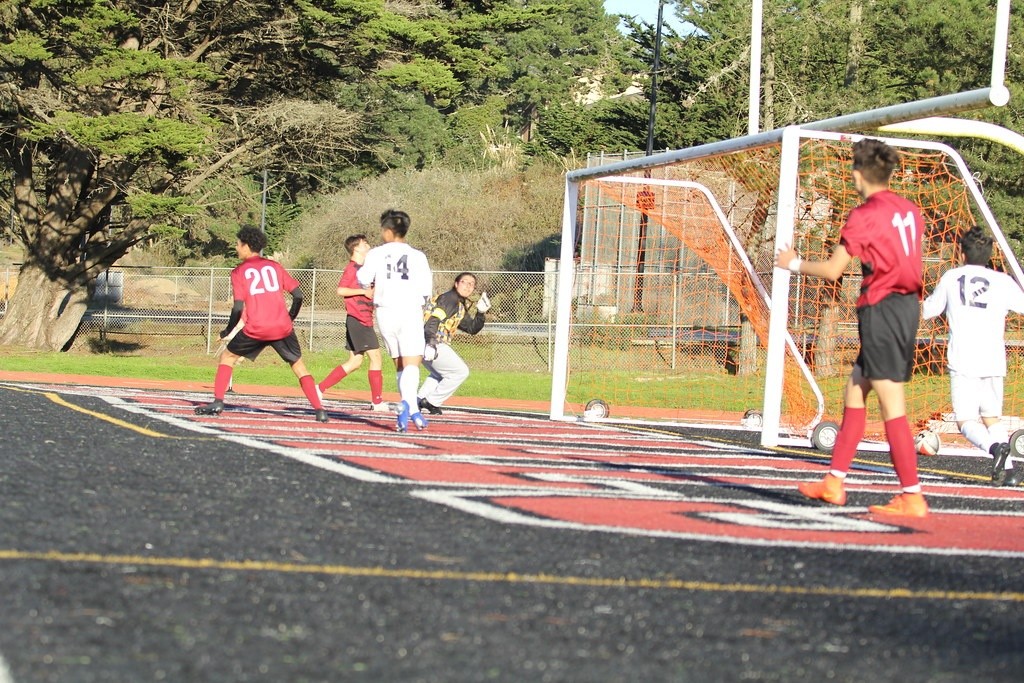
[914,427,942,458]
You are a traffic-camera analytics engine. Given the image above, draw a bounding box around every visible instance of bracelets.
[788,257,802,274]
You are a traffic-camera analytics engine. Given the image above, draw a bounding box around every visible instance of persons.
[918,222,1024,488]
[192,226,333,425]
[356,211,429,433]
[313,232,398,414]
[769,137,928,520]
[413,271,491,418]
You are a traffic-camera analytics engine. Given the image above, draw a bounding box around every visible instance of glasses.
[459,280,476,288]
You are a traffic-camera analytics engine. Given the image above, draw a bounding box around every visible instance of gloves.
[423,344,436,361]
[476,292,491,313]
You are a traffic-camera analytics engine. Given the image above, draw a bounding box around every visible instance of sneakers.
[418,398,442,415]
[315,384,323,402]
[394,400,410,433]
[989,442,1011,487]
[371,401,389,412]
[1002,470,1018,487]
[316,408,330,423]
[798,474,847,505]
[194,400,224,415]
[410,411,428,431]
[868,492,929,516]
